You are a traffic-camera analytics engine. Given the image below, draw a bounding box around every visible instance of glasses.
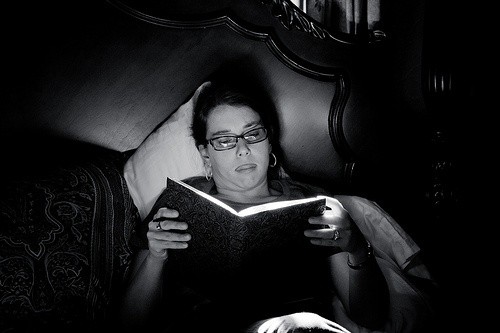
[208,125,269,151]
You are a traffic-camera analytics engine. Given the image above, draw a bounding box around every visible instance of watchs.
[347,240,374,269]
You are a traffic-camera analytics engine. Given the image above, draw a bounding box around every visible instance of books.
[166,175,326,266]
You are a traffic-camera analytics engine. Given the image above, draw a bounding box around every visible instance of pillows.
[122,81,292,222]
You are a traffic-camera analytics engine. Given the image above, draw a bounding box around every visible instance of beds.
[0,0,391,333]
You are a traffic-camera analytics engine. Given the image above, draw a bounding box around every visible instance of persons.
[125,80,390,333]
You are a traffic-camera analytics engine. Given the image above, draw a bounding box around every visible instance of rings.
[334,230,340,241]
[156,221,162,231]
